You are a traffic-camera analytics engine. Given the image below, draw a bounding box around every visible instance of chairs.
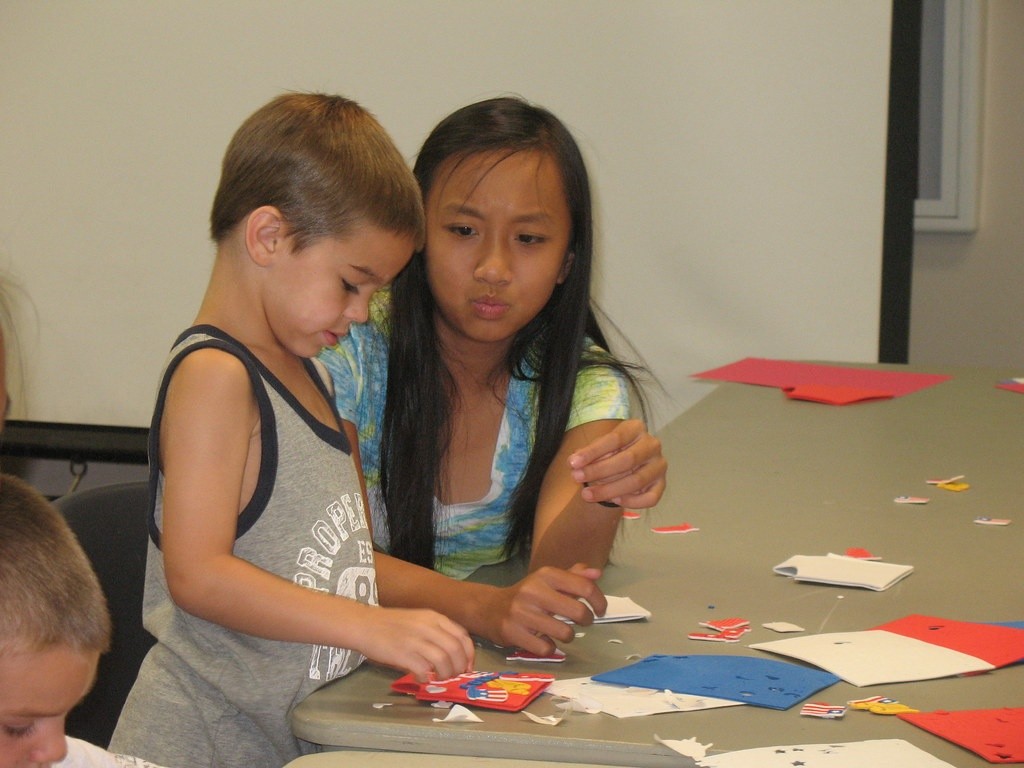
[44,468,162,744]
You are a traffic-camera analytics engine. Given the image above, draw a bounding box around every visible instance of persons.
[1,472,165,767]
[311,96,668,660]
[102,91,475,768]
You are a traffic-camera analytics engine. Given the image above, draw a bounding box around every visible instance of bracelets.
[583,481,621,508]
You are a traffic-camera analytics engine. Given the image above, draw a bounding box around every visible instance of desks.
[274,350,1024,768]
